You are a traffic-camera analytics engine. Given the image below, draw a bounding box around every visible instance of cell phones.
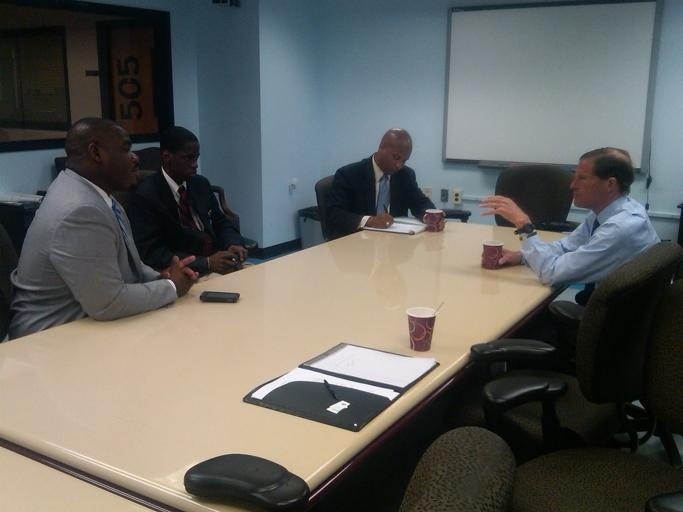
[200,291,239,304]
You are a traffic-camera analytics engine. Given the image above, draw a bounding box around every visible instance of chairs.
[398,424,682,509]
[493,165,579,233]
[315,174,348,239]
[208,186,258,254]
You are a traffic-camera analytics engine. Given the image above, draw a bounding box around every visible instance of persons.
[478,146,661,377]
[8,116,200,340]
[129,126,256,284]
[326,127,445,241]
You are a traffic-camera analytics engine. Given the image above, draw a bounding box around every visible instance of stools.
[440,209,472,224]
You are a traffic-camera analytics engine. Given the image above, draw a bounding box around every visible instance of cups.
[483,241,504,269]
[425,209,443,232]
[407,307,437,351]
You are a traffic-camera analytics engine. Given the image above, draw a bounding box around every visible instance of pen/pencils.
[383,204,388,214]
[324,378,339,403]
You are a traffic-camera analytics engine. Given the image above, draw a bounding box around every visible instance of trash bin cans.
[298,206,328,250]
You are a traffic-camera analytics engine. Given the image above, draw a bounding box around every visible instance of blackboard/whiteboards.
[442,0,664,179]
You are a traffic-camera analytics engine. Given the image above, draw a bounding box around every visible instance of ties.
[377,174,390,218]
[177,185,200,232]
[112,199,128,245]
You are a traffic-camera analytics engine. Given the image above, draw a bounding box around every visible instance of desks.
[0,447,169,512]
[0,218,571,511]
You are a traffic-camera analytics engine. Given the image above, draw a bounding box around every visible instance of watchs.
[514,222,536,235]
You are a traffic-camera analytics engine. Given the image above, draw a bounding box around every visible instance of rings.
[386,222,390,226]
